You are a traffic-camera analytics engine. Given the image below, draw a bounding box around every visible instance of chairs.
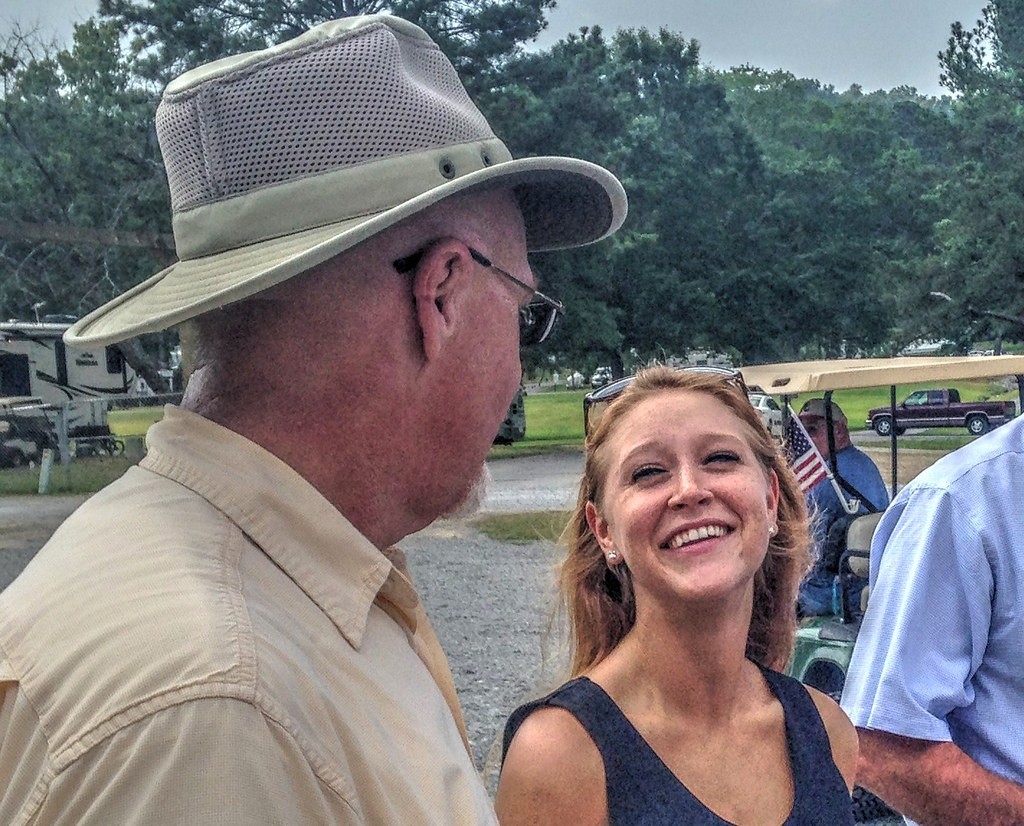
[838,510,886,624]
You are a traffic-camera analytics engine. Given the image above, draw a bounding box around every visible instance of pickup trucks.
[865,387,1016,437]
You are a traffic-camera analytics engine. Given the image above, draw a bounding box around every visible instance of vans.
[592,366,613,388]
[566,370,584,389]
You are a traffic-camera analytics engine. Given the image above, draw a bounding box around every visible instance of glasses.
[583,365,752,440]
[393,240,564,343]
[807,423,827,437]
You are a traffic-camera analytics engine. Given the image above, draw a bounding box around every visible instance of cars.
[967,350,995,357]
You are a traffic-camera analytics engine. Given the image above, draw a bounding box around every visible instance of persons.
[796,398,891,621]
[494,360,861,826]
[0,11,629,826]
[837,411,1023,825]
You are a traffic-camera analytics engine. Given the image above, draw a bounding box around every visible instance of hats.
[63,15,628,347]
[797,399,849,425]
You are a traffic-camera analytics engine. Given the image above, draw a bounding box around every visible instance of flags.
[783,402,828,494]
